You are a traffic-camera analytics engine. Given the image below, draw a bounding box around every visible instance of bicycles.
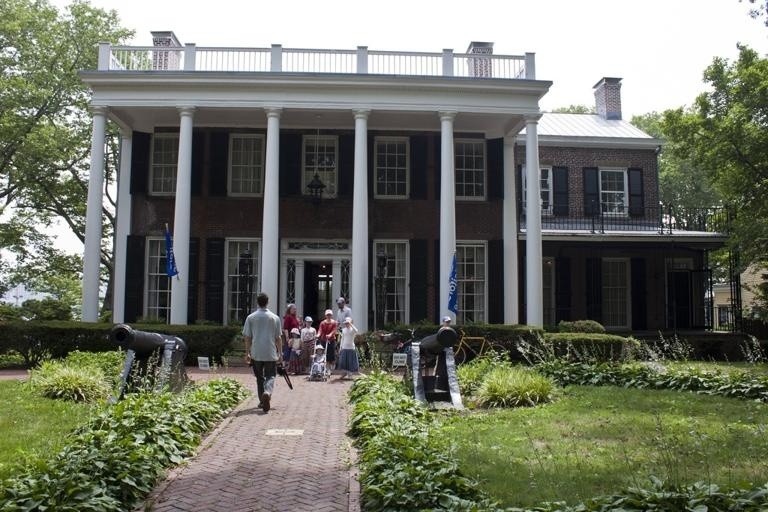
[380,328,416,372]
[452,328,507,367]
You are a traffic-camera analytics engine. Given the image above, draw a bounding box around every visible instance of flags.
[165,225,178,276]
[447,252,458,315]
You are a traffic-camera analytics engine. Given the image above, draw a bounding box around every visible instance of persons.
[299,315,317,374]
[310,344,326,377]
[441,315,452,374]
[282,305,300,370]
[336,297,352,333]
[286,327,301,375]
[241,292,283,411]
[314,309,339,371]
[336,317,361,378]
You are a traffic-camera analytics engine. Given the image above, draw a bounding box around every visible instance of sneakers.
[261,392,272,413]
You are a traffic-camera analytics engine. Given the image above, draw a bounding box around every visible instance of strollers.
[309,339,328,382]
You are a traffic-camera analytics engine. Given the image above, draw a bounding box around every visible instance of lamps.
[307,113,326,212]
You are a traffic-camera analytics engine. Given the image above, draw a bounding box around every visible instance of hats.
[442,316,452,323]
[305,316,313,323]
[290,327,301,336]
[335,297,345,304]
[343,316,353,325]
[324,309,334,317]
[315,344,325,351]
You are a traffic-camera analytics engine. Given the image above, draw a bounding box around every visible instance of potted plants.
[221,351,251,367]
